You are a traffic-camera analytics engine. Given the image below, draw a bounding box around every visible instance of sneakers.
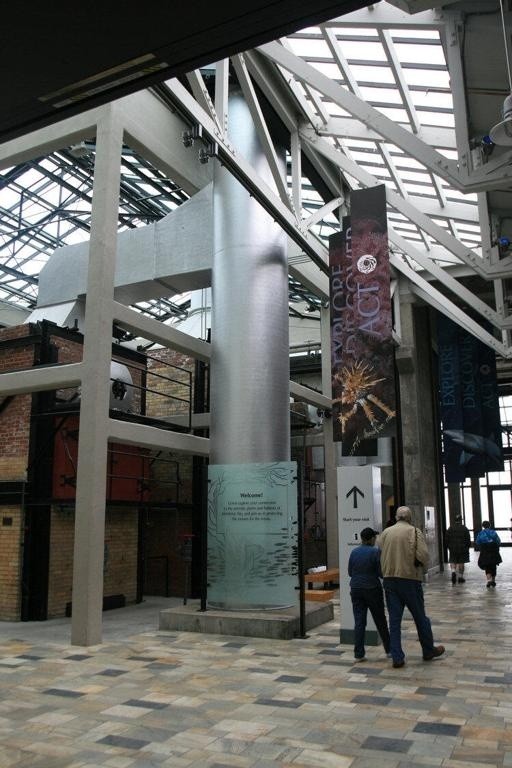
[385,650,404,668]
[450,574,496,588]
[422,644,446,661]
[352,653,369,663]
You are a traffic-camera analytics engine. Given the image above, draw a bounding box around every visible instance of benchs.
[305,568,339,589]
[304,590,334,601]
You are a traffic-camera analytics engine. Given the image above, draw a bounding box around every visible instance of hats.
[359,526,380,540]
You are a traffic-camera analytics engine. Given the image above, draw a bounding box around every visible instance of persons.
[378,506,445,668]
[444,514,471,584]
[347,528,392,663]
[475,521,502,588]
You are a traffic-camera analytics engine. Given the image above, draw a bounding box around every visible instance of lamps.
[489,93,512,147]
